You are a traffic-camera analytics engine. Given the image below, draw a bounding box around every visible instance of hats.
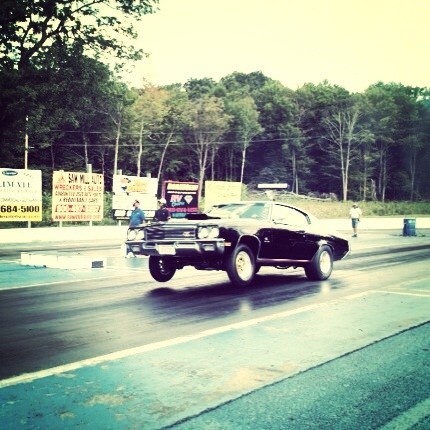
[157,198,166,203]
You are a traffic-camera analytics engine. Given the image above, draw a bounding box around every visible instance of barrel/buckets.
[404,219,416,237]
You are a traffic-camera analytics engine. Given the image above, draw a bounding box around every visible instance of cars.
[126,199,349,287]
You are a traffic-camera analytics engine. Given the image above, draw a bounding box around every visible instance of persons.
[124,200,147,258]
[152,198,169,220]
[348,204,359,238]
